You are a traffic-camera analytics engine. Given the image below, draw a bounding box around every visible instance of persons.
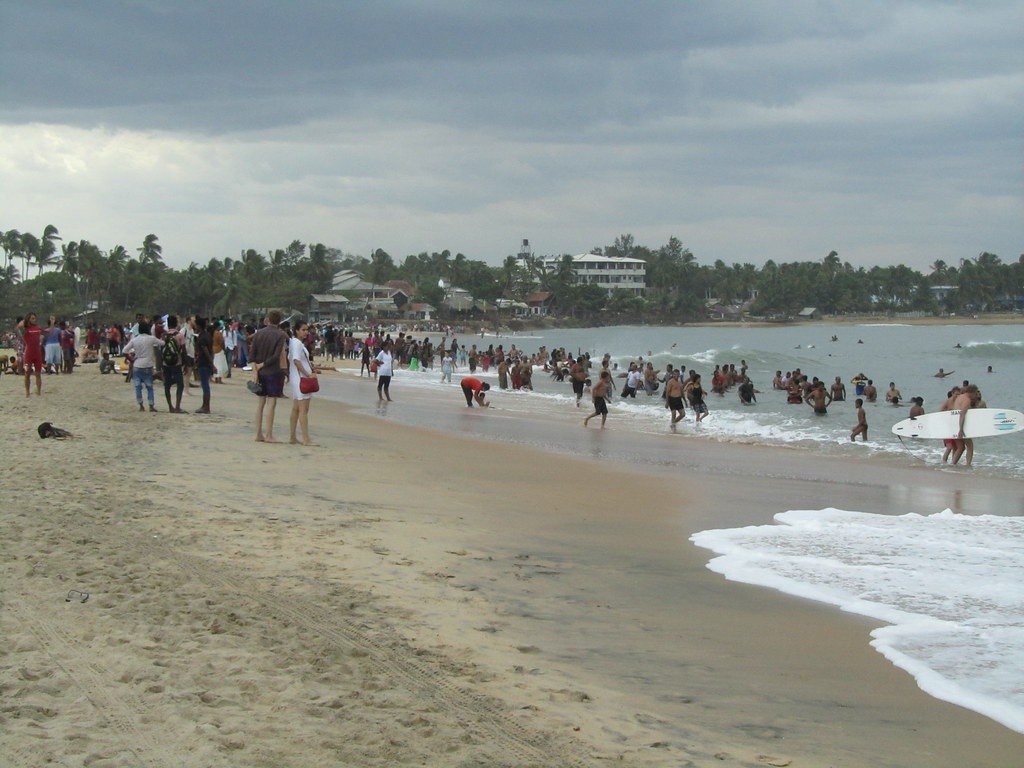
[935,369,954,377]
[0,312,709,429]
[850,399,868,442]
[830,377,846,401]
[287,321,320,445]
[738,377,757,405]
[773,368,826,404]
[851,374,877,401]
[938,380,986,466]
[806,381,832,413]
[248,310,286,443]
[988,366,992,372]
[910,396,924,417]
[18,312,55,398]
[374,342,394,401]
[584,371,612,428]
[954,344,961,348]
[712,360,759,393]
[886,382,902,404]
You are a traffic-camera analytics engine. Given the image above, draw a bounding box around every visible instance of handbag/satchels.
[300,377,319,394]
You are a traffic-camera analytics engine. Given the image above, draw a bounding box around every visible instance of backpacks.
[161,331,182,365]
